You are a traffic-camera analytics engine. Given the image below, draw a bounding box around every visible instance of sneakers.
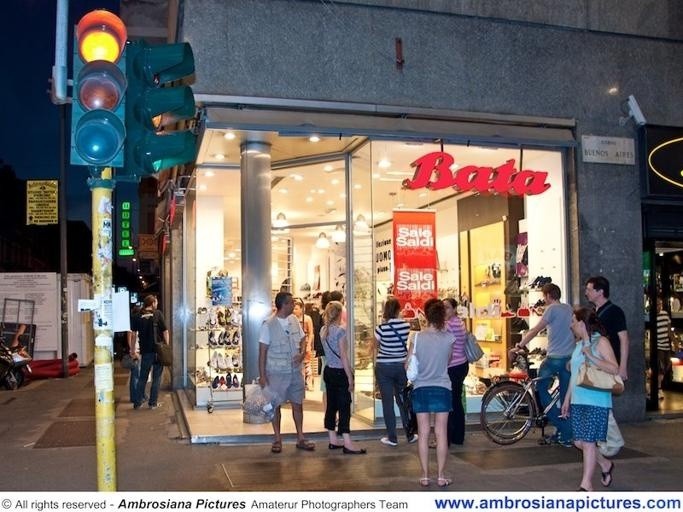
[197,306,240,329]
[526,347,547,362]
[380,436,397,448]
[209,350,239,372]
[131,394,162,411]
[210,372,240,391]
[528,275,552,288]
[407,432,419,445]
[207,330,217,346]
[218,331,231,346]
[231,329,240,346]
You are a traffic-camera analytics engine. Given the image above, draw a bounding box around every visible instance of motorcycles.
[0,336,32,391]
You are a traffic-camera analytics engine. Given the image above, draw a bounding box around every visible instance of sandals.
[295,439,315,451]
[418,477,431,488]
[270,440,282,452]
[436,477,453,487]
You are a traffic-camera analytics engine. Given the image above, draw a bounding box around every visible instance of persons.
[130,295,170,410]
[319,300,367,454]
[257,292,316,453]
[374,296,469,487]
[507,274,630,492]
[645,295,672,399]
[314,291,344,427]
[127,302,150,403]
[291,296,314,398]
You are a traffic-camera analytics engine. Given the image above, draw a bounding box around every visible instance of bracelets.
[301,352,306,357]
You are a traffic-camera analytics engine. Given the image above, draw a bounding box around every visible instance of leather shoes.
[342,446,367,456]
[327,442,344,450]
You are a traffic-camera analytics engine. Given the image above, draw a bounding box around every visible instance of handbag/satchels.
[154,343,173,366]
[575,361,625,396]
[405,331,419,383]
[463,330,484,365]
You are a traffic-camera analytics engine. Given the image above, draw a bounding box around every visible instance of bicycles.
[477,342,561,447]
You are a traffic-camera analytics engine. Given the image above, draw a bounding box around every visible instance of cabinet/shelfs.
[188,310,243,414]
[502,287,549,359]
[470,281,502,370]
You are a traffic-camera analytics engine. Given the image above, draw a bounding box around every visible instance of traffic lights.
[125,34,204,176]
[66,4,132,172]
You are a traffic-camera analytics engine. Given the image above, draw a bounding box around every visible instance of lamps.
[272,212,368,249]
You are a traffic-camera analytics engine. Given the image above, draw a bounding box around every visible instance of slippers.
[600,461,614,488]
[573,486,590,491]
